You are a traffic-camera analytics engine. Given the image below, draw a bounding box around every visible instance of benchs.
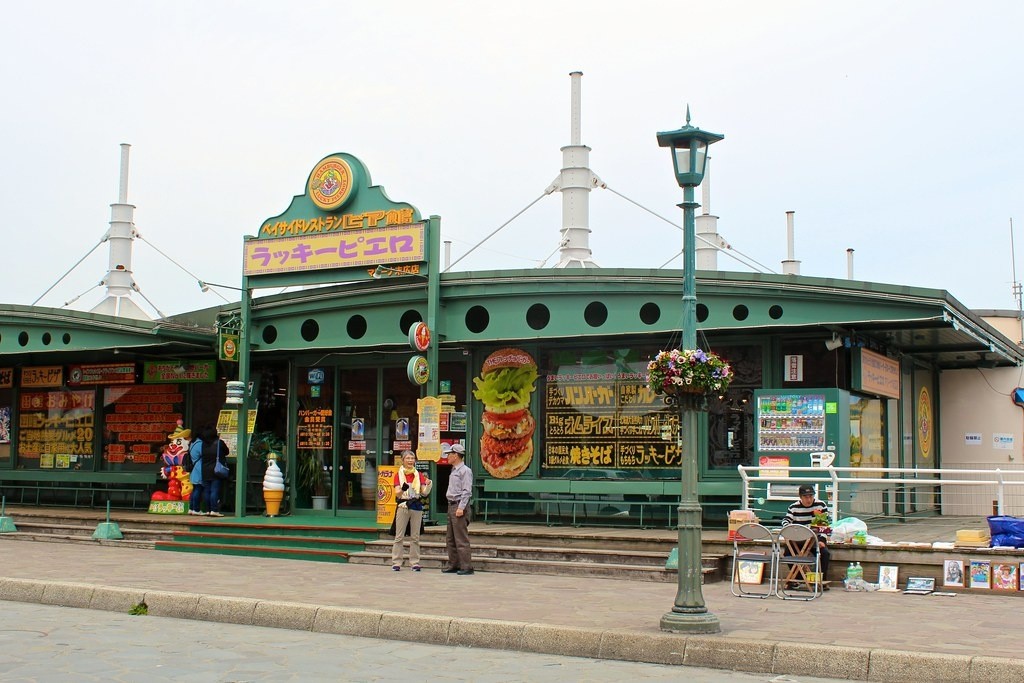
[474,479,753,534]
[0,470,157,512]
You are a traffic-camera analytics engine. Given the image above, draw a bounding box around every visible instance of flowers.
[646,348,735,394]
[812,509,829,526]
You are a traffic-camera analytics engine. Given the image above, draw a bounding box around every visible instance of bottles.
[761,395,824,448]
[855,562,863,579]
[846,563,857,579]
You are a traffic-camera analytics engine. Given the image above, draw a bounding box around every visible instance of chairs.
[731,523,823,601]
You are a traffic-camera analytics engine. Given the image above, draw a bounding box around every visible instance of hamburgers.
[472,349,541,478]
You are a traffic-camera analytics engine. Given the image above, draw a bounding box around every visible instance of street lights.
[656,100,722,636]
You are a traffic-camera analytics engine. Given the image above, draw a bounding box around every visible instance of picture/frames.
[942,558,966,588]
[968,558,991,589]
[878,563,899,589]
[907,576,936,591]
[991,561,1019,591]
[734,550,766,585]
[1019,562,1024,591]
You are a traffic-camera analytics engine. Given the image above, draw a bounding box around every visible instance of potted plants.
[296,394,329,510]
[249,428,286,474]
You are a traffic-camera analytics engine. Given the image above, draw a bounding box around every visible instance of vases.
[818,523,825,526]
[661,385,709,411]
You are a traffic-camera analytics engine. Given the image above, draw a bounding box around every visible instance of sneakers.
[411,564,421,571]
[392,565,400,571]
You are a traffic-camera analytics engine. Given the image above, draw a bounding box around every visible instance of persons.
[781,484,831,591]
[389,451,432,571]
[201,425,229,517]
[442,444,475,575]
[188,425,206,515]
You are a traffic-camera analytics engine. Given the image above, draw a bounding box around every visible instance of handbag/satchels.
[213,461,230,480]
[406,499,423,511]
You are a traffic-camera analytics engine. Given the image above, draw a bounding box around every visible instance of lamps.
[199,280,253,293]
[373,265,429,280]
[172,359,190,375]
[824,331,843,351]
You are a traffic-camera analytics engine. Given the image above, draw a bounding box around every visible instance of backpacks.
[182,440,203,472]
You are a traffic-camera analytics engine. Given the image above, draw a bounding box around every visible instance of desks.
[770,530,832,591]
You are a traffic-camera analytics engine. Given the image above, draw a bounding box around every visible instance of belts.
[447,499,460,506]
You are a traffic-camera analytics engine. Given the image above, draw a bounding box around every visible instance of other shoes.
[814,584,830,592]
[457,568,474,575]
[192,510,204,516]
[188,509,193,515]
[209,512,224,517]
[793,580,802,587]
[207,511,211,516]
[442,567,461,573]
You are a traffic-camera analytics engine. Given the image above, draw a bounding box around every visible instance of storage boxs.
[851,535,866,544]
[727,517,761,542]
[954,529,990,547]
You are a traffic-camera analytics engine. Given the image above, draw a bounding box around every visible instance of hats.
[444,443,466,455]
[798,485,816,496]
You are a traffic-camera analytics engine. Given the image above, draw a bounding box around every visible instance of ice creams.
[361,460,377,510]
[263,460,285,515]
